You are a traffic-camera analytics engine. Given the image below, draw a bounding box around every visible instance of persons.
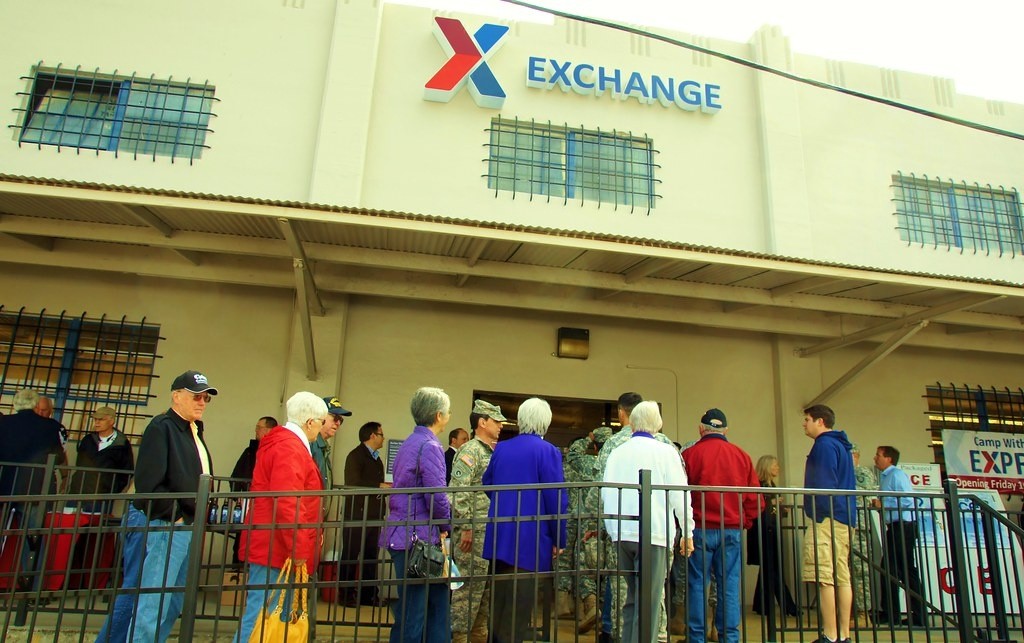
[378,387,452,643]
[232,391,328,643]
[802,404,857,643]
[229,417,278,572]
[308,397,353,643]
[445,392,766,643]
[126,370,217,643]
[339,422,394,607]
[746,455,804,617]
[848,442,881,628]
[0,389,148,643]
[870,446,928,626]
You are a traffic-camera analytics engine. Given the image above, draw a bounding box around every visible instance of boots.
[849,610,871,628]
[551,590,584,619]
[453,618,488,643]
[578,594,602,634]
[707,605,718,641]
[670,606,685,635]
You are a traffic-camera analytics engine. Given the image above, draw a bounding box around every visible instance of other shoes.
[789,607,804,616]
[339,596,387,608]
[812,634,852,643]
[870,614,927,626]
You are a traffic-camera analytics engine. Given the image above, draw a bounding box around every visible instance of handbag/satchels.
[248,557,309,643]
[409,539,445,579]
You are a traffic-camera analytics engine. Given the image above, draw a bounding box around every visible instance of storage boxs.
[221,573,249,607]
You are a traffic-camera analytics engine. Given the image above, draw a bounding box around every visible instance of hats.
[473,400,507,421]
[593,427,612,442]
[850,443,861,453]
[14,390,39,411]
[171,370,218,395]
[90,407,115,419]
[701,408,727,428]
[323,397,352,416]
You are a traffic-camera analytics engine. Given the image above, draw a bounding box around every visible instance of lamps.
[551,327,589,360]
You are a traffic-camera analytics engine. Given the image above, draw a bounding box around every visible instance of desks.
[0,513,122,603]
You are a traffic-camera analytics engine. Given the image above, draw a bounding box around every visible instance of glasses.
[318,418,326,425]
[376,434,383,437]
[178,391,211,402]
[329,413,343,425]
[256,425,266,429]
